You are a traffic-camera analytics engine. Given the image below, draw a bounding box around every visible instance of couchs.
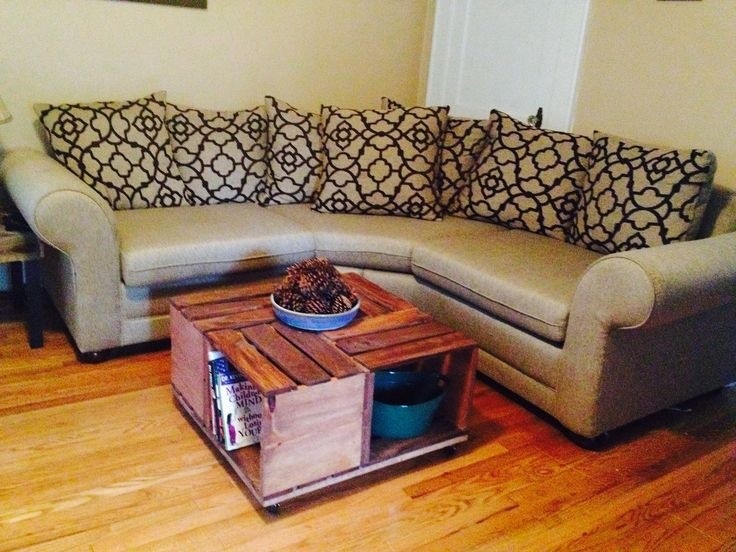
[0,121,736,447]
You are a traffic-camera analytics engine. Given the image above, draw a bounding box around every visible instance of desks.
[168,273,480,510]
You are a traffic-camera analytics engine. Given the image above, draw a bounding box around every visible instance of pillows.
[382,98,491,209]
[254,96,327,205]
[448,108,590,242]
[32,93,193,208]
[570,131,716,256]
[310,105,451,220]
[162,100,278,206]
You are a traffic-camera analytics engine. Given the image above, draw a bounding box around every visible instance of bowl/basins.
[369,369,447,440]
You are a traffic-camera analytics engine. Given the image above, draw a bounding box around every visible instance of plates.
[271,290,362,332]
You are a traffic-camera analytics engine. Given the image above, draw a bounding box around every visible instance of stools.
[0,209,46,348]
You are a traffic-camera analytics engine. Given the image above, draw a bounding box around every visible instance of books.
[208,350,263,452]
